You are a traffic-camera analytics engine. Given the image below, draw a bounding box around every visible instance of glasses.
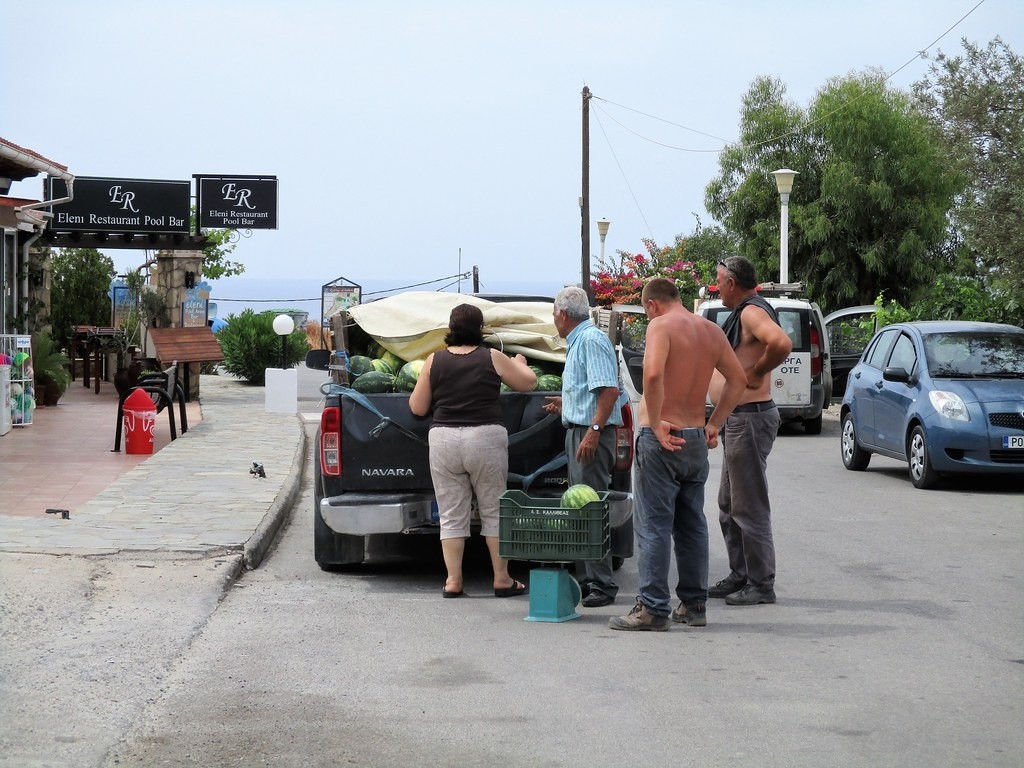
[718,259,740,278]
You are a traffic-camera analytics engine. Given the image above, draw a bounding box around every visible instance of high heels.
[443,584,464,598]
[494,579,527,597]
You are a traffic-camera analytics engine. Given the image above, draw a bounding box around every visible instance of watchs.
[590,424,602,433]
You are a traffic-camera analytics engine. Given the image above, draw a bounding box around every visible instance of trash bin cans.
[122,388,157,455]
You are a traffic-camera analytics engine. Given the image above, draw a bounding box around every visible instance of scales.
[522,559,582,623]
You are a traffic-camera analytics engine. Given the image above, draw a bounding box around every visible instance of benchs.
[111,360,188,452]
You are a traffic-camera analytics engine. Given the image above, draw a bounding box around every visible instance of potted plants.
[34,329,74,407]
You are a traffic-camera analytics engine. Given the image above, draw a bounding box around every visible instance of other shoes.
[582,591,615,607]
[576,581,590,598]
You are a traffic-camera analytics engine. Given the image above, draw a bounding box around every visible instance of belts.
[568,422,581,429]
[732,399,776,413]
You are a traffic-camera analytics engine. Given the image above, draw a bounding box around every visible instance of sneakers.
[672,602,707,626]
[608,603,670,631]
[725,584,777,605]
[708,577,746,598]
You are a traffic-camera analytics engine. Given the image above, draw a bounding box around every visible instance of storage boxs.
[498,490,611,562]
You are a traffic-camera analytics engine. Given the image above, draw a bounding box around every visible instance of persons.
[708,255,793,605]
[608,277,748,632]
[540,285,625,607]
[409,304,539,600]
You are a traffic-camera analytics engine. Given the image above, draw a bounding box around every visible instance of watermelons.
[500,364,562,392]
[348,329,425,394]
[512,483,600,554]
